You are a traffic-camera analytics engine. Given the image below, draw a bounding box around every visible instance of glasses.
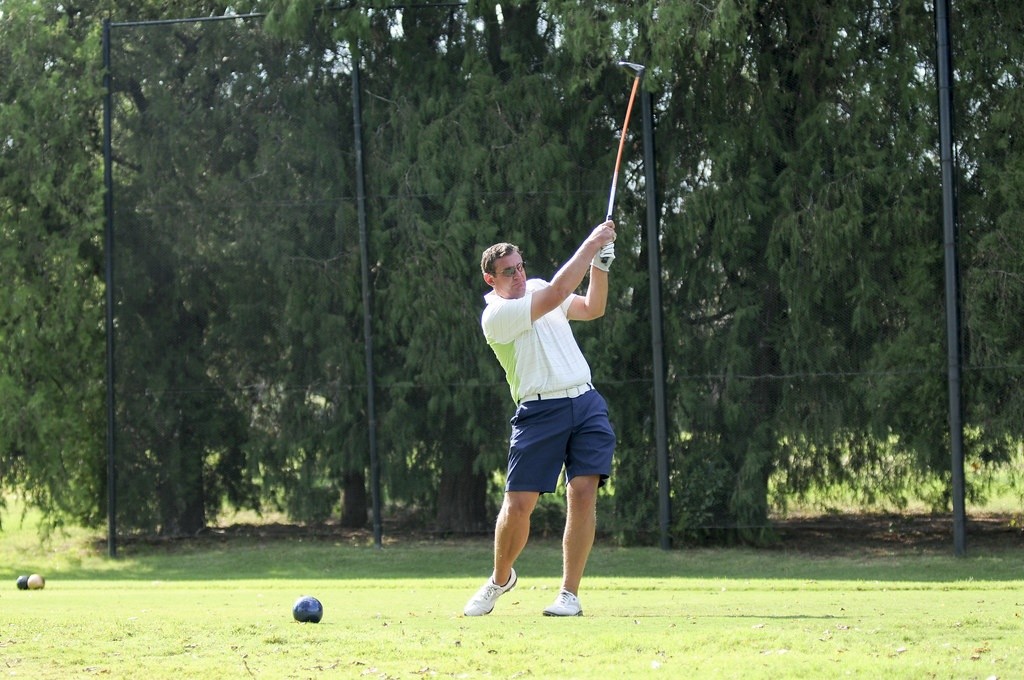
[490,260,527,277]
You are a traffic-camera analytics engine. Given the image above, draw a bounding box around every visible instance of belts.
[518,383,595,406]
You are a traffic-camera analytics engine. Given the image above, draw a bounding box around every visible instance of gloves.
[590,241,616,272]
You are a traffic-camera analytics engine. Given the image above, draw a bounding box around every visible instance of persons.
[463,219,618,619]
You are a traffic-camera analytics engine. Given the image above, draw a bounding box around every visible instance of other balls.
[27,574,45,589]
[292,596,323,623]
[16,576,29,590]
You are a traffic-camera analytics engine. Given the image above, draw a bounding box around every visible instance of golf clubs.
[601,62,645,263]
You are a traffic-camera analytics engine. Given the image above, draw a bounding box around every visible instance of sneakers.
[543,589,583,616]
[464,566,517,616]
